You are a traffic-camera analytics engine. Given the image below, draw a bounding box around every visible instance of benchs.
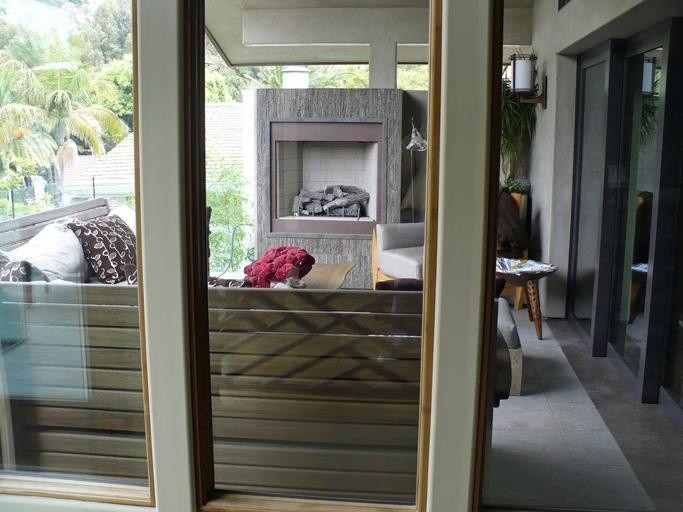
[1,198,512,512]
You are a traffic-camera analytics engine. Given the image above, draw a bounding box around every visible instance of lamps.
[641,55,656,96]
[406,117,427,221]
[509,50,547,109]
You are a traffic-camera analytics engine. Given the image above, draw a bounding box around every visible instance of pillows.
[1,203,137,282]
[375,278,422,291]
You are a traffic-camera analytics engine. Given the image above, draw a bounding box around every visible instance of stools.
[493,294,524,396]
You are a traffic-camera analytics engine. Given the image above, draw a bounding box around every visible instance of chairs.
[495,190,530,312]
[371,221,423,290]
[628,190,652,324]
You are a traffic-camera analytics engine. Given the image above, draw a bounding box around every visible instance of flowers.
[244,245,315,291]
